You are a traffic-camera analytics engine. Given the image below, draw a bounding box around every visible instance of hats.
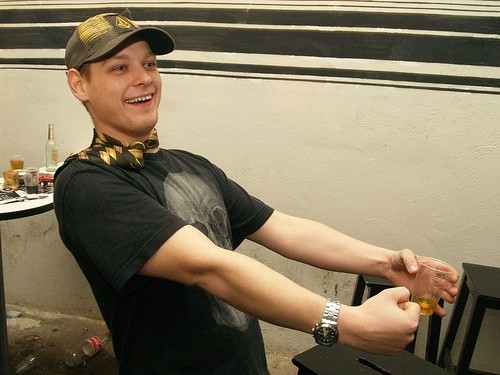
[64,13,176,71]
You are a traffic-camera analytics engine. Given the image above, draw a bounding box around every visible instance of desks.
[0,178,54,375]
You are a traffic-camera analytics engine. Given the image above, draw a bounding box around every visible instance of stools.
[292,342,456,375]
[350,273,444,364]
[437,263,500,375]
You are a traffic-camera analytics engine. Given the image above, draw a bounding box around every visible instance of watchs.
[313,298,342,346]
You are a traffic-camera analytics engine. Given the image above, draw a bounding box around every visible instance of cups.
[408,258,457,316]
[3,169,20,190]
[24,169,39,194]
[10,154,24,170]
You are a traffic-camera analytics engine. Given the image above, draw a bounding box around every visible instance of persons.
[53,13,459,375]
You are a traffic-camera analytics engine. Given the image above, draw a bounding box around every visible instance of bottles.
[46,124,58,172]
